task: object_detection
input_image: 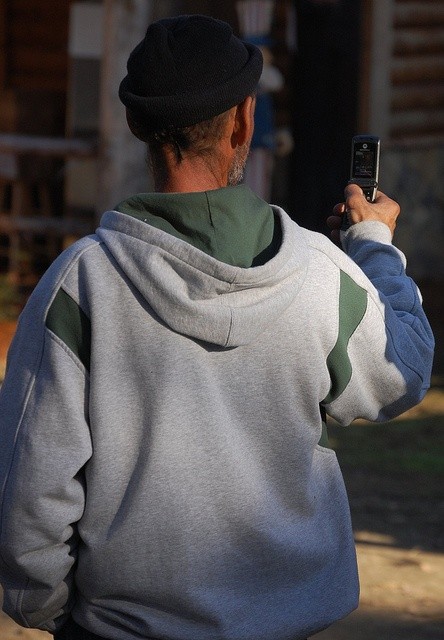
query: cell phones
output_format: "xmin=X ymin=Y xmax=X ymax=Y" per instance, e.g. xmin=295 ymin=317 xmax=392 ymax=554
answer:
xmin=342 ymin=136 xmax=381 ymax=232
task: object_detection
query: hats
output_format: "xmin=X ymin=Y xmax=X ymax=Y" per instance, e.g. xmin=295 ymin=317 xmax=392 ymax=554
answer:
xmin=118 ymin=15 xmax=264 ymax=126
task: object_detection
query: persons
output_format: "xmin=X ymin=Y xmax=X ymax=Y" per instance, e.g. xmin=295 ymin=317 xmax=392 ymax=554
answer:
xmin=0 ymin=12 xmax=436 ymax=638
